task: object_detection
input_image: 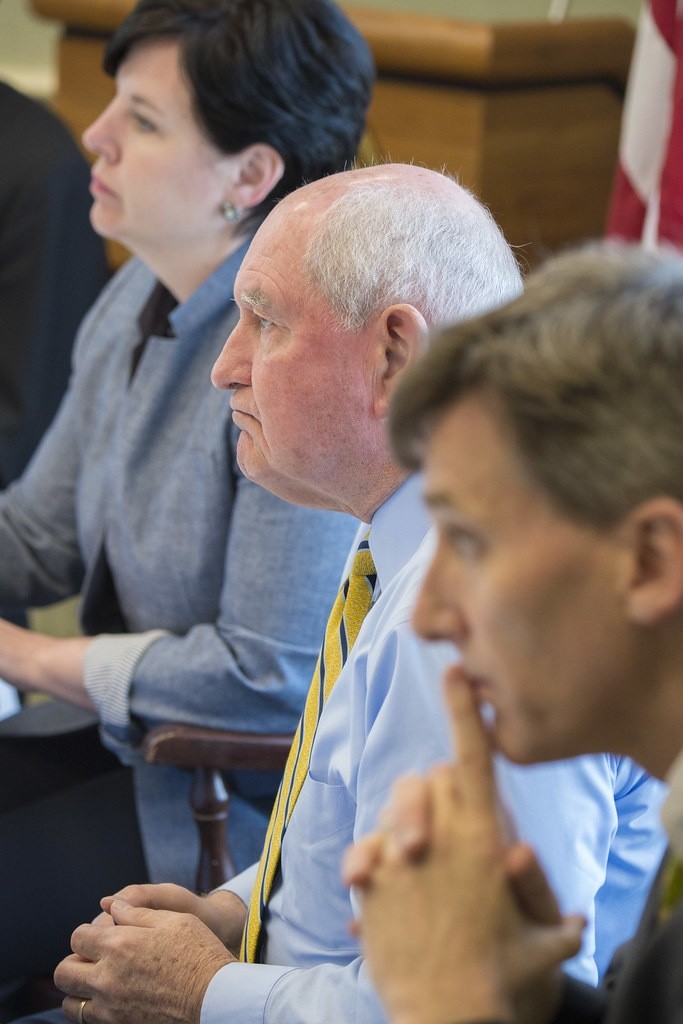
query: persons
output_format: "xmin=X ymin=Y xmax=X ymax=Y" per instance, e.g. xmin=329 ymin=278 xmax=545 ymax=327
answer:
xmin=338 ymin=238 xmax=683 ymax=1024
xmin=54 ymin=163 xmax=668 ymax=1024
xmin=0 ymin=0 xmax=377 ymax=1022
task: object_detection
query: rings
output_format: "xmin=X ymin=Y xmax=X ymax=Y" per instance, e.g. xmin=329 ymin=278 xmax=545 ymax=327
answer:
xmin=77 ymin=999 xmax=87 ymax=1024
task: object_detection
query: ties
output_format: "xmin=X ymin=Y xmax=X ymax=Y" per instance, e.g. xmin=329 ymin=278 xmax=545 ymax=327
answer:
xmin=233 ymin=542 xmax=379 ymax=961
